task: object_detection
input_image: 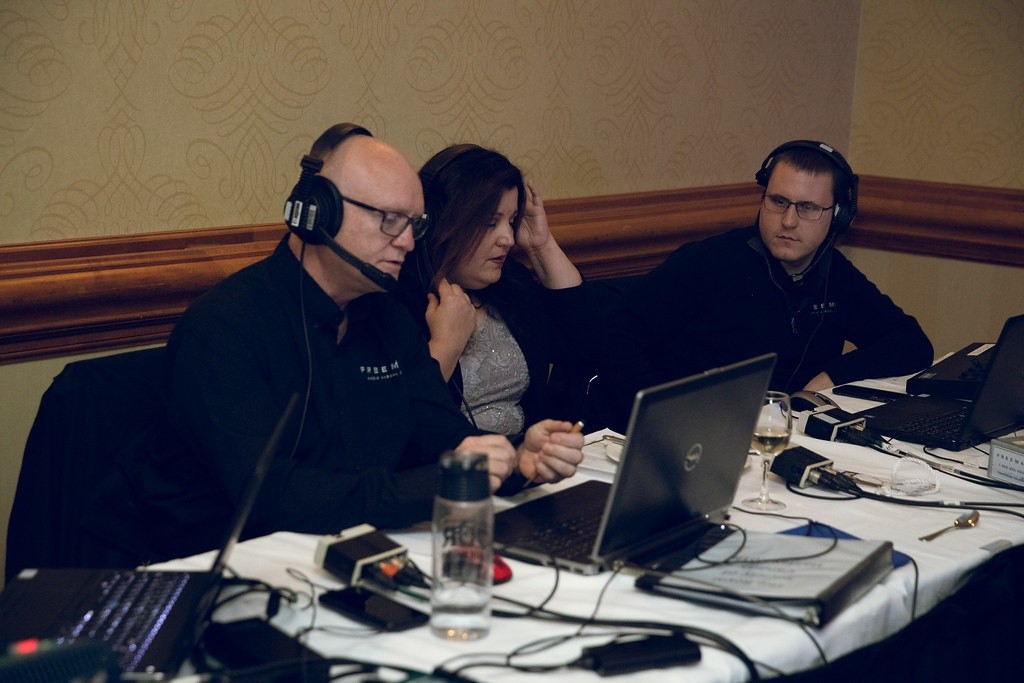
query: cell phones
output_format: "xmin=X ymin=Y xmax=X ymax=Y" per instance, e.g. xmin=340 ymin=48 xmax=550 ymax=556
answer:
xmin=319 ymin=584 xmax=429 ymax=632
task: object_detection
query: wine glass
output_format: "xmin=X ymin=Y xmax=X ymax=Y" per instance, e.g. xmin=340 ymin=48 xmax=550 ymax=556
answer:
xmin=741 ymin=390 xmax=793 ymax=513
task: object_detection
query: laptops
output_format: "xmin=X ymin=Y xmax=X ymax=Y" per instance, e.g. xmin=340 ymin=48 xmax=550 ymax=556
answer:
xmin=492 ymin=352 xmax=777 ymax=577
xmin=854 ymin=313 xmax=1024 ymax=451
xmin=0 ymin=398 xmax=304 ymax=682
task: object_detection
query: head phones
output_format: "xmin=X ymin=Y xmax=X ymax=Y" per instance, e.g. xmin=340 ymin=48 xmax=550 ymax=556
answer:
xmin=756 ymin=141 xmax=861 ymax=236
xmin=284 ymin=121 xmax=372 ymax=245
xmin=410 ymin=142 xmax=477 ymax=241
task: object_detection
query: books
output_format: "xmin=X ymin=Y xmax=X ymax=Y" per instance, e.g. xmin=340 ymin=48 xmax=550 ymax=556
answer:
xmin=635 ymin=527 xmax=893 ymax=632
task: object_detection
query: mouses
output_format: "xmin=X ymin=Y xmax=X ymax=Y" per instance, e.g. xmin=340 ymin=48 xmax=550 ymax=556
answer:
xmin=790 ymin=390 xmax=841 ymax=418
xmin=444 ymin=545 xmax=512 ymax=585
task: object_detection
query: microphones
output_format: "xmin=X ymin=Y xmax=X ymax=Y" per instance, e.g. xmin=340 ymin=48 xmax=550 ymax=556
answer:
xmin=318 ymin=226 xmax=398 ymax=292
xmin=421 ymin=240 xmax=440 ymax=300
xmin=792 ymin=231 xmax=838 ymax=285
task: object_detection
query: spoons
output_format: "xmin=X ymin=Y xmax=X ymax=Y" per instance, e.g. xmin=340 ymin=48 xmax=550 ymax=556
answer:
xmin=918 ymin=510 xmax=980 ymax=543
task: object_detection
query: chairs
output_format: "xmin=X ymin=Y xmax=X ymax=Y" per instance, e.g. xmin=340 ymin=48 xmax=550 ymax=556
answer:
xmin=3 ymin=347 xmax=183 ymax=594
xmin=545 ymin=273 xmax=646 ymax=436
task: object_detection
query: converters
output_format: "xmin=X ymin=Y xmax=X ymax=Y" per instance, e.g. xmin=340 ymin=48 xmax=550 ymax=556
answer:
xmin=202 ymin=616 xmax=330 ymax=682
xmin=582 ymin=636 xmax=701 ymax=676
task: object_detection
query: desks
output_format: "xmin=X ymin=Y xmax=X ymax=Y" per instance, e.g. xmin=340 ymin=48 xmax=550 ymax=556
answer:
xmin=133 ymin=350 xmax=1024 ymax=683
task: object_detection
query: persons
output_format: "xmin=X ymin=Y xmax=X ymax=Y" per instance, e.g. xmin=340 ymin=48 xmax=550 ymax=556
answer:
xmin=650 ymin=140 xmax=935 ymax=398
xmin=365 ymin=145 xmax=647 ymax=447
xmin=126 ymin=134 xmax=584 ymax=552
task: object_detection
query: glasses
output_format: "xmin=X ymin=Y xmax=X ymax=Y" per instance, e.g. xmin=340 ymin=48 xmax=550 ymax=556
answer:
xmin=761 ymin=187 xmax=834 ymax=220
xmin=342 ymin=195 xmax=429 ymax=240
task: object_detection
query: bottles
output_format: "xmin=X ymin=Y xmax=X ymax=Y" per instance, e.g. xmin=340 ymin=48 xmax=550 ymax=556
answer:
xmin=428 ymin=450 xmax=494 ymax=643
